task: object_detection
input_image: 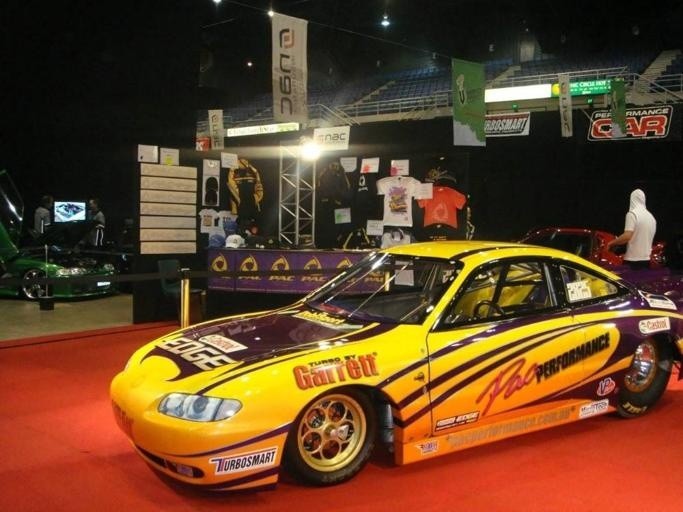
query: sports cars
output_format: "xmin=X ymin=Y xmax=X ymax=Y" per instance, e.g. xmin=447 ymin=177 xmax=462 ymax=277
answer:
xmin=105 ymin=239 xmax=682 ymax=494
xmin=0 ymin=169 xmax=119 ymax=301
xmin=501 ymin=226 xmax=667 ymax=275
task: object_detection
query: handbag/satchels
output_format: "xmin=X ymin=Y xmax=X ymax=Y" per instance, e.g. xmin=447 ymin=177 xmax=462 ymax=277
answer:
xmin=381 ymin=228 xmax=410 ymax=250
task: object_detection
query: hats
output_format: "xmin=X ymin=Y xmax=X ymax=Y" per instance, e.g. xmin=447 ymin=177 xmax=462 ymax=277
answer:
xmin=225 ymin=234 xmax=244 ymax=249
xmin=208 ymin=235 xmax=225 ymax=248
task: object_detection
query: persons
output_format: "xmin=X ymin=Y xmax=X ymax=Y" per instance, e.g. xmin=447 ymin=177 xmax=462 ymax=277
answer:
xmin=33 ymin=194 xmax=54 ymax=233
xmin=605 ymin=187 xmax=657 ymax=270
xmin=82 ymin=198 xmax=105 ymax=248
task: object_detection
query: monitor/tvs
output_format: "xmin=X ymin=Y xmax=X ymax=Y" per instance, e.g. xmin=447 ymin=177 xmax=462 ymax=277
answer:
xmin=54 ymin=201 xmax=87 ymax=223
